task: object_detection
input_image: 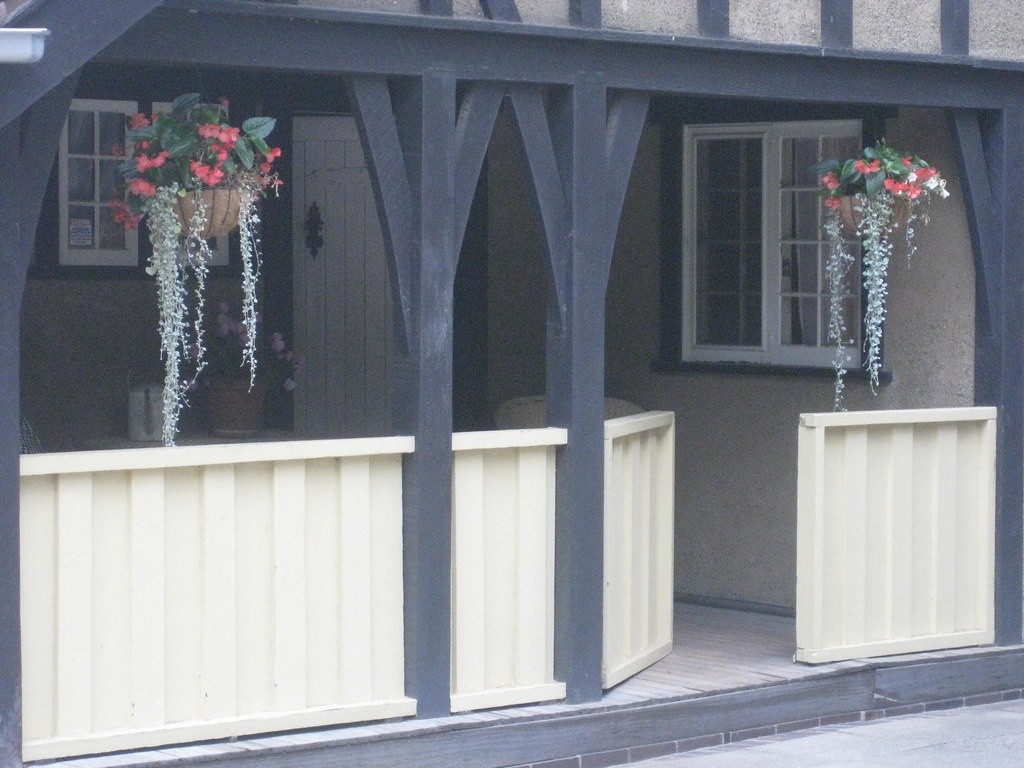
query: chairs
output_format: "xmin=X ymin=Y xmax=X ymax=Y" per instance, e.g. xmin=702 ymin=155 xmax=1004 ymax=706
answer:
xmin=493 ymin=395 xmax=647 ymax=431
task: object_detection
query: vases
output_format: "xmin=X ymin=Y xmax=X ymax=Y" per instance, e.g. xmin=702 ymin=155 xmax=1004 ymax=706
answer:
xmin=166 ymin=187 xmax=249 ymax=239
xmin=837 ymin=192 xmax=912 ymax=242
xmin=203 ymin=374 xmax=274 ymax=440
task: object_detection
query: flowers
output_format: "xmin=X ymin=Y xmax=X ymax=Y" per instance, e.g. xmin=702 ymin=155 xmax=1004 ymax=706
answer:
xmin=105 ymin=91 xmax=284 ymax=447
xmin=807 ymin=137 xmax=949 ymax=413
xmin=182 ymin=309 xmax=307 ymax=393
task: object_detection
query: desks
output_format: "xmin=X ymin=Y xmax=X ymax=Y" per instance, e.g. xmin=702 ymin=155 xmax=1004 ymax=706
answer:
xmin=86 ymin=425 xmax=316 ymax=451
